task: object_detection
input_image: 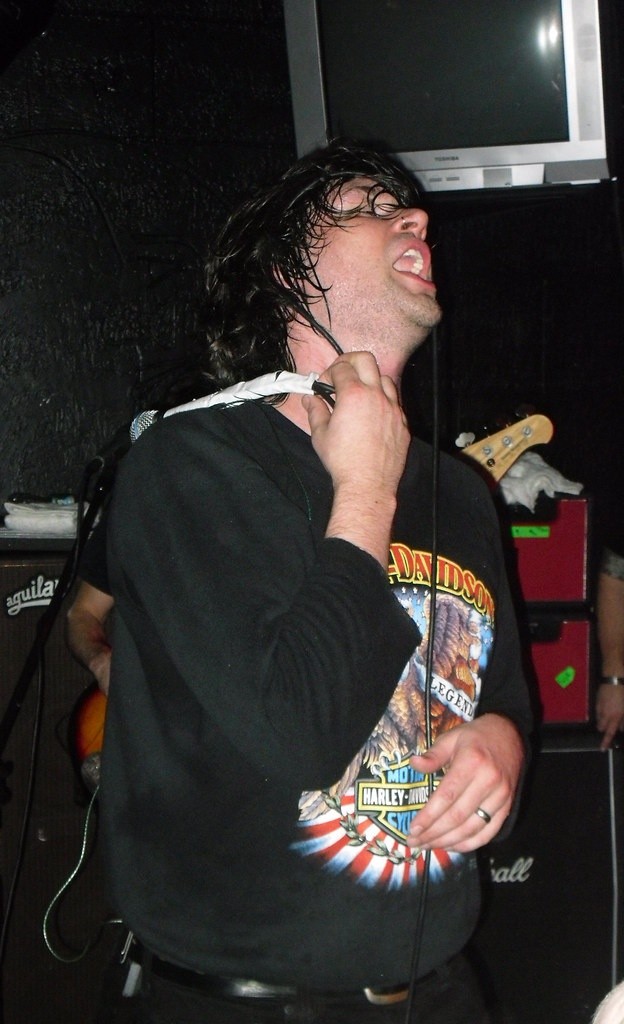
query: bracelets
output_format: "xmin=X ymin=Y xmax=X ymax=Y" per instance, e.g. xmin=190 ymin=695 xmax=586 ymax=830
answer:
xmin=600 ymin=677 xmax=624 ymax=685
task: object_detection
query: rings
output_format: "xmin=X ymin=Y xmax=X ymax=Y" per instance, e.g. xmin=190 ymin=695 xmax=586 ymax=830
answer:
xmin=475 ymin=808 xmax=491 ymax=824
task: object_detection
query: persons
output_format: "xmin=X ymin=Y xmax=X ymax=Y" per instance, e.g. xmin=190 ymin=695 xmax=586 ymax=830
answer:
xmin=66 ymin=137 xmax=544 ymax=1024
xmin=594 ymin=492 xmax=624 ymax=753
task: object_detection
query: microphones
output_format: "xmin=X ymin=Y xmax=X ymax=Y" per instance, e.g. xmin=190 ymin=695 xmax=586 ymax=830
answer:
xmin=89 ymin=423 xmax=132 ymax=473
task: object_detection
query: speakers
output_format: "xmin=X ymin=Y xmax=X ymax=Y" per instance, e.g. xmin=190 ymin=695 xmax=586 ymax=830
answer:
xmin=0 ymin=528 xmax=148 ymax=1024
xmin=466 ymin=722 xmax=624 ymax=1024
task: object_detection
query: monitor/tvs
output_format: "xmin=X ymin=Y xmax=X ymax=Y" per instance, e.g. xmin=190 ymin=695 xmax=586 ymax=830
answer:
xmin=284 ymin=0 xmax=624 ymax=194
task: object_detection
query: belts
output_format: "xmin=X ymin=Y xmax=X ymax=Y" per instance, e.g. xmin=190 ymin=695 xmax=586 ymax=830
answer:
xmin=127 ymin=944 xmax=438 ymax=1007
